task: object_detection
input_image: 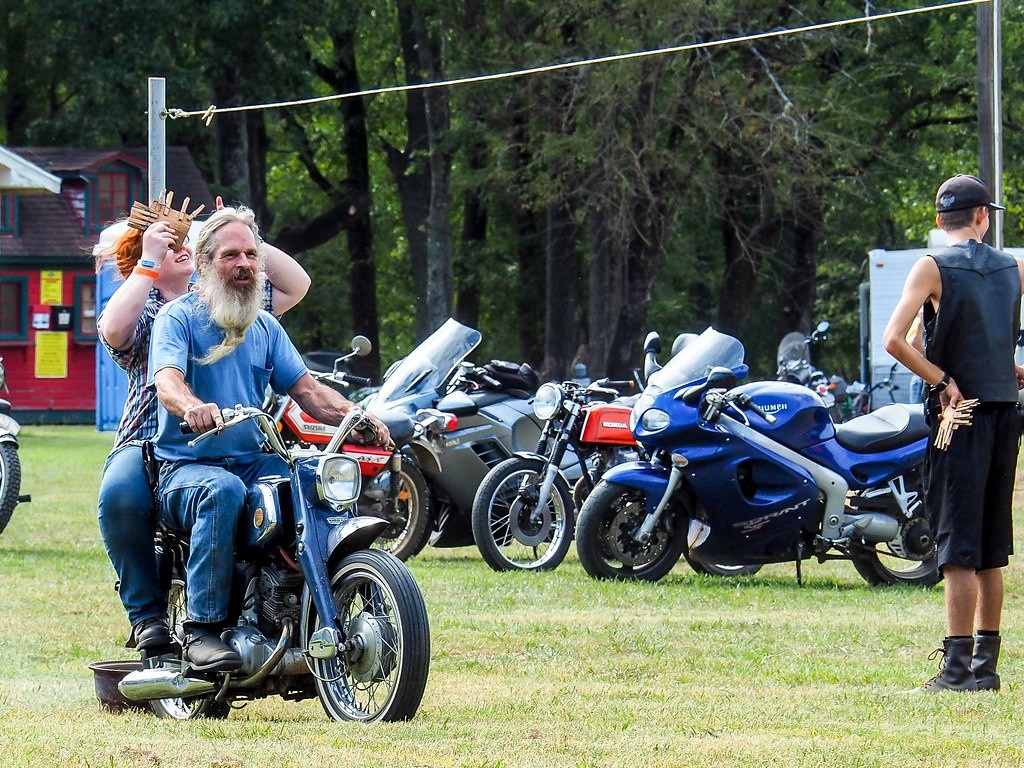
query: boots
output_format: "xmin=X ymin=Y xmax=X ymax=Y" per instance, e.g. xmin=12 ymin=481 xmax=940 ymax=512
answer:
xmin=894 ymin=636 xmax=1002 ymax=697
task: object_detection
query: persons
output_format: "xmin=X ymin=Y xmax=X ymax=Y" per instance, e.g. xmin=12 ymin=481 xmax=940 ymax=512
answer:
xmin=883 ymin=174 xmax=1024 ymax=695
xmin=97 ymin=195 xmax=311 ymax=650
xmin=146 ymin=207 xmax=395 ymax=669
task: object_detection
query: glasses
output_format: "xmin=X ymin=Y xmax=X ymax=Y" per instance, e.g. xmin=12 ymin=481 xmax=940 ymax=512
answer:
xmin=166 ymin=235 xmax=190 ymax=250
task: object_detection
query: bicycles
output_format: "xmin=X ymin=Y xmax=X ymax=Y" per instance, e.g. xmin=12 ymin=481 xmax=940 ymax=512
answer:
xmin=845 ymin=362 xmax=901 ymax=418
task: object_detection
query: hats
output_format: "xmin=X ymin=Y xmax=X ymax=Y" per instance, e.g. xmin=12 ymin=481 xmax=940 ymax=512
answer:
xmin=935 ymin=174 xmax=1006 ymax=213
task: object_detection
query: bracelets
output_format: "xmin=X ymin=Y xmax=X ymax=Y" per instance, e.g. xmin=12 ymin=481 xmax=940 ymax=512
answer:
xmin=937 ymin=373 xmax=950 ymax=392
xmin=133 ymin=266 xmax=159 ymax=281
xmin=137 ymin=259 xmax=161 ymax=268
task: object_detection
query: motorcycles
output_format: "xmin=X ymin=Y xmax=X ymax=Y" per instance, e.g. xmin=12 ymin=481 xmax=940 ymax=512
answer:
xmin=775 ymin=322 xmax=842 ymax=425
xmin=261 ymin=317 xmax=644 ymax=563
xmin=575 ymin=325 xmax=944 ymax=590
xmin=470 ymin=377 xmax=766 ymax=578
xmin=0 ymin=358 xmax=31 ymax=535
xmin=117 ymin=403 xmax=431 ymax=724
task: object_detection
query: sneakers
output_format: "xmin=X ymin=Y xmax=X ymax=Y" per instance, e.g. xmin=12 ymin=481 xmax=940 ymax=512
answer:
xmin=125 ymin=617 xmax=170 ymax=652
xmin=181 ymin=620 xmax=243 ymax=678
